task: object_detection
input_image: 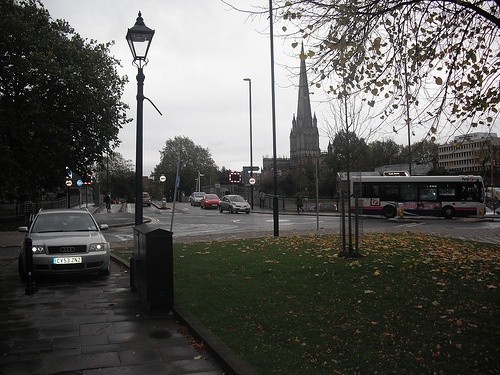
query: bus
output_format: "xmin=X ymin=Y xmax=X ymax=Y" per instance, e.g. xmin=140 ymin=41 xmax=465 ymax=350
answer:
xmin=348 ymin=170 xmax=486 ymax=218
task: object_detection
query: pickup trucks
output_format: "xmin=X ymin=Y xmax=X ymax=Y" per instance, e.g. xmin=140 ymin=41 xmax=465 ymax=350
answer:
xmin=141 ymin=191 xmax=151 ymax=205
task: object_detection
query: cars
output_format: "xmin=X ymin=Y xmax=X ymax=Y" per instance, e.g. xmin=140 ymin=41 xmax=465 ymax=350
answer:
xmin=201 ymin=193 xmax=220 ymax=209
xmin=17 ymin=206 xmax=112 ymax=283
xmin=219 ymin=195 xmax=251 ymax=214
xmin=190 ymin=192 xmax=206 ymax=206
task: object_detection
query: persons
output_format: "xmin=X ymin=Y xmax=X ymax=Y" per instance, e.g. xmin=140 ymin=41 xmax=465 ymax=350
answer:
xmin=296 ymin=194 xmax=304 ymax=214
xmin=259 ymin=190 xmax=265 ymax=208
xmin=106 ymin=193 xmax=112 ymax=213
xmin=23 ymin=197 xmax=33 ymax=228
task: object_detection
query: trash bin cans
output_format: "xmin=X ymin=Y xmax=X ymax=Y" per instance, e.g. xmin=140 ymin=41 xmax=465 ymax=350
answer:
xmin=162 ymin=197 xmax=166 ymax=207
xmin=129 ymin=222 xmax=174 ymax=305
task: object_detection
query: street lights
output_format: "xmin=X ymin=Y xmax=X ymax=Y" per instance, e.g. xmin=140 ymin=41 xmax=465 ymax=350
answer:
xmin=126 ymin=9 xmax=156 ymax=289
xmin=243 ymin=77 xmax=254 ymax=209
